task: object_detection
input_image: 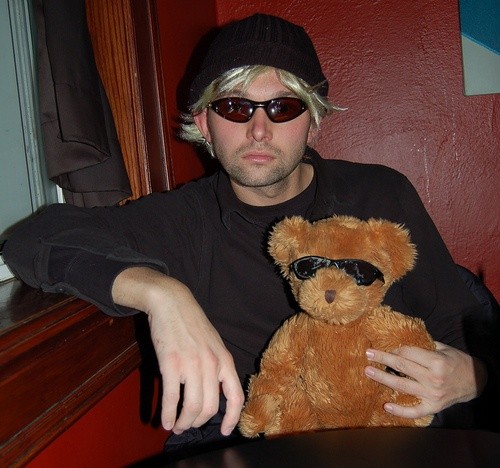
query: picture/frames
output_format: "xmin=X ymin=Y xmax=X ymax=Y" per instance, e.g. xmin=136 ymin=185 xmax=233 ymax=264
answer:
xmin=457 ymin=0 xmax=500 ymax=96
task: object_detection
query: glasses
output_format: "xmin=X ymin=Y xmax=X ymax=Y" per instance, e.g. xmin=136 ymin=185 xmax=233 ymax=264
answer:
xmin=207 ymin=97 xmax=308 ymax=123
xmin=289 ymin=256 xmax=387 ymax=287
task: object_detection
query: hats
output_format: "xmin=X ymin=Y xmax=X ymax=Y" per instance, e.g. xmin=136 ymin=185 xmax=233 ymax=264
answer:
xmin=177 ymin=14 xmax=328 ymax=114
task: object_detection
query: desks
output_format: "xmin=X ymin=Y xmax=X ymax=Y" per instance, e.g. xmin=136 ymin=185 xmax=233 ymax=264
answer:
xmin=123 ymin=427 xmax=500 ymax=467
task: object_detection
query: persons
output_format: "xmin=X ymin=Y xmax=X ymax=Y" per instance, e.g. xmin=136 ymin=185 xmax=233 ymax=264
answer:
xmin=0 ymin=13 xmax=499 ymax=449
